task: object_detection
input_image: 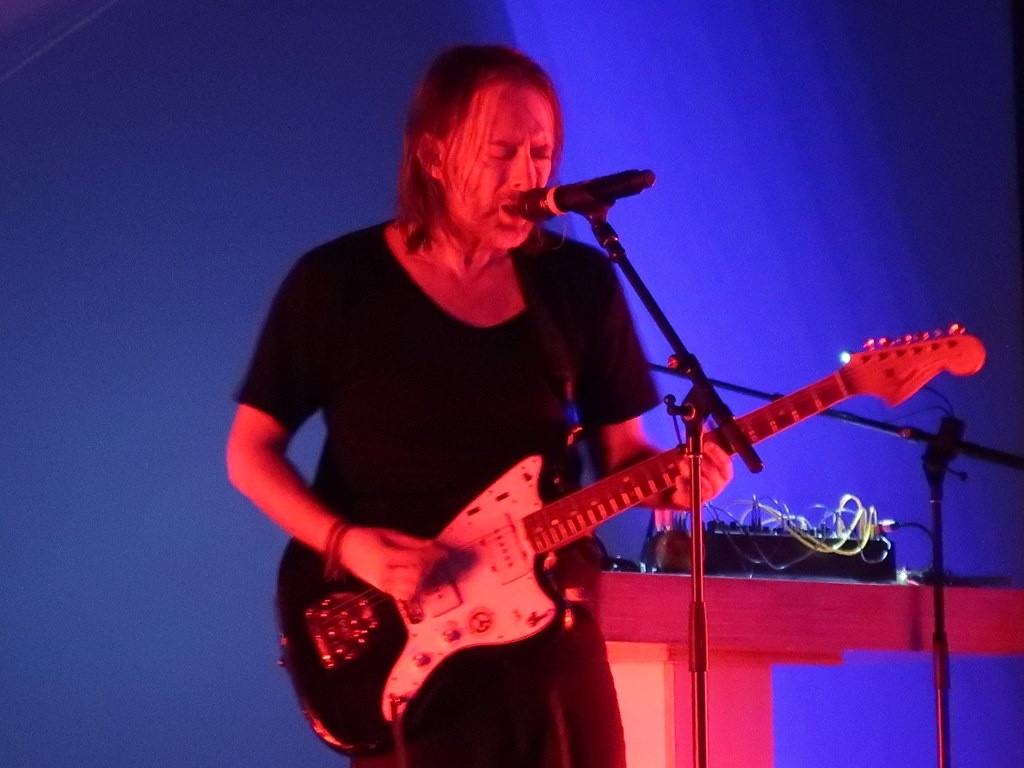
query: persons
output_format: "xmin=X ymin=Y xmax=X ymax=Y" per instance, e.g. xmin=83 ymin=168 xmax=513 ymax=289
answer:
xmin=226 ymin=41 xmax=735 ymax=768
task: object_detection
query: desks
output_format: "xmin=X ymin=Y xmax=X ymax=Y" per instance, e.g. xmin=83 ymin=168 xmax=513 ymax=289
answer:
xmin=597 ymin=570 xmax=1024 ymax=768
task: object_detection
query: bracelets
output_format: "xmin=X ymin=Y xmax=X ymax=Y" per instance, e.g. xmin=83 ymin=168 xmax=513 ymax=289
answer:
xmin=322 ymin=518 xmax=353 ymax=562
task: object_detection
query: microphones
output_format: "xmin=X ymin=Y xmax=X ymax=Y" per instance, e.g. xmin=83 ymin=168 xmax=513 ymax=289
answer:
xmin=518 ymin=169 xmax=657 ymax=224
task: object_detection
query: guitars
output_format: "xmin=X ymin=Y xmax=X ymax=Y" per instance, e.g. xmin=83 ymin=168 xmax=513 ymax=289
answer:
xmin=274 ymin=324 xmax=988 ymax=755
